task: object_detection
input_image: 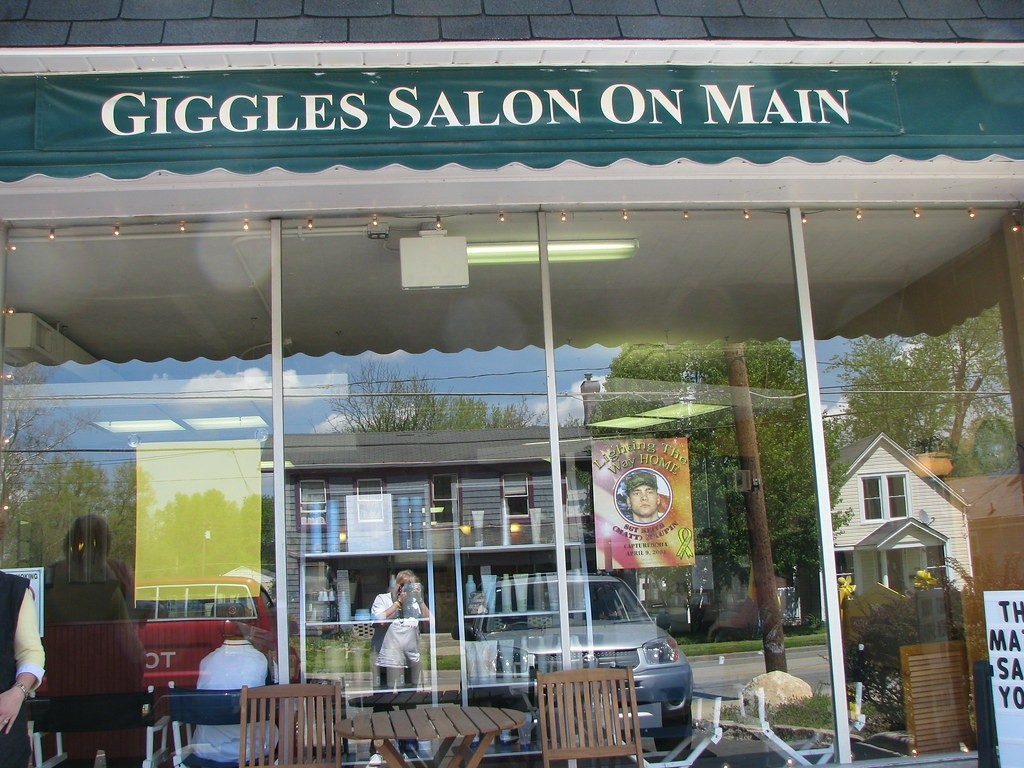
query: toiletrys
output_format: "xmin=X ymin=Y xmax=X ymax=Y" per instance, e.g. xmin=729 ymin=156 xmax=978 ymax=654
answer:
xmin=464 ymin=497 xmax=584 ymax=752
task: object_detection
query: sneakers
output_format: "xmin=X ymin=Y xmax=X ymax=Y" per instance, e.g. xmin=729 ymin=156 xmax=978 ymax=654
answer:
xmin=369 ymin=750 xmax=385 ymax=765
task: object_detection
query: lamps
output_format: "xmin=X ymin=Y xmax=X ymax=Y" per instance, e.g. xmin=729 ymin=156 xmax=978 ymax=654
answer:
xmin=468 ymin=238 xmax=641 ymax=265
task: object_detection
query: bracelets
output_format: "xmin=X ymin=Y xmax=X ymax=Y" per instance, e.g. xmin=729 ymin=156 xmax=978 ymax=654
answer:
xmin=417 ymin=598 xmax=423 ymax=604
xmin=394 ymin=599 xmax=402 ymax=608
xmin=14 ymin=682 xmax=29 ymax=701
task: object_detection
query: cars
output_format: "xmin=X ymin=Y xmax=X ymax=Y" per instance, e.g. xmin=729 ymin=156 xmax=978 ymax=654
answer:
xmin=451 ymin=576 xmax=692 ymax=762
xmin=124 ymin=577 xmax=299 ymax=749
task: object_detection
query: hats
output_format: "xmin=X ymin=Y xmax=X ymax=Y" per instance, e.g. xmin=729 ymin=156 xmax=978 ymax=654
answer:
xmin=626 ymin=472 xmax=658 ymax=491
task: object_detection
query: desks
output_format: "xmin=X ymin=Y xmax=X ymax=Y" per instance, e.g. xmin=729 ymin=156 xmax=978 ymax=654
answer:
xmin=336 ymin=705 xmax=527 ymax=768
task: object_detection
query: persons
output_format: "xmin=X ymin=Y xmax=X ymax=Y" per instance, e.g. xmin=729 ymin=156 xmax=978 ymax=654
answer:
xmin=0 ymin=510 xmax=45 ymax=768
xmin=366 ymin=569 xmax=430 ymax=766
xmin=41 ymin=514 xmax=150 ymax=768
xmin=180 ymin=600 xmax=279 ymax=768
xmin=626 ymin=473 xmax=661 ymax=524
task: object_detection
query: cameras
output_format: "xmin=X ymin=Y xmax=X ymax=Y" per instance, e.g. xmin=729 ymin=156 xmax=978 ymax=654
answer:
xmin=403 ymin=583 xmax=417 ymax=592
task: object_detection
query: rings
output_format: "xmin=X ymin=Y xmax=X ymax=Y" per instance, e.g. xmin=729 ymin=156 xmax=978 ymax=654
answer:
xmin=2 ymin=720 xmax=8 ymax=725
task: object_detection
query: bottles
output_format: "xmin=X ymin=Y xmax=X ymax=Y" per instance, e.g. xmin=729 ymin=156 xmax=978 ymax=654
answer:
xmin=466 ymin=575 xmax=477 ymax=615
xmin=519 ymin=635 xmax=530 ymax=678
xmin=306 ymin=590 xmax=340 ymax=622
xmin=533 ymin=573 xmax=545 ymax=611
xmin=573 ymin=569 xmax=585 ymax=610
xmin=538 ymin=636 xmax=548 ymax=673
xmin=501 ymin=574 xmax=512 ymax=614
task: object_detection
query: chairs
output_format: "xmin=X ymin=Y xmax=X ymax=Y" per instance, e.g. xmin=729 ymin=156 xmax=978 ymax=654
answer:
xmin=535 ymin=667 xmax=644 ymax=768
xmin=239 ymin=683 xmax=342 ymax=767
xmin=167 ymin=680 xmax=278 ymax=768
xmin=25 ymin=685 xmax=171 ymax=768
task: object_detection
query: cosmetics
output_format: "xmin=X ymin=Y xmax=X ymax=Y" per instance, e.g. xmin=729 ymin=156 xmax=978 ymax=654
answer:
xmin=354 ymin=609 xmax=369 ymax=620
xmin=464 ymin=497 xmax=588 ymax=755
